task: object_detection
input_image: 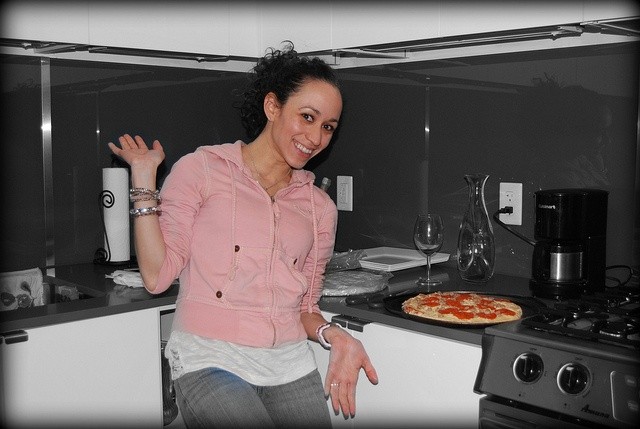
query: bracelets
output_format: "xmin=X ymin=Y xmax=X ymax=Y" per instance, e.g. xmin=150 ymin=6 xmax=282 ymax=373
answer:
xmin=129 ymin=206 xmax=162 ymax=217
xmin=129 ymin=187 xmax=161 ymax=204
xmin=316 ymin=321 xmax=337 ymax=349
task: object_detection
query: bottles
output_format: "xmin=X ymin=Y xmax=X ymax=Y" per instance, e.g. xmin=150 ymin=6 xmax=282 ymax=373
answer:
xmin=456 ymin=172 xmax=496 ymax=283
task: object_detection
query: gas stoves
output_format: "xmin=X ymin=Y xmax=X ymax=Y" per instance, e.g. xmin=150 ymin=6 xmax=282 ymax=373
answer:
xmin=471 ymin=281 xmax=639 ymax=429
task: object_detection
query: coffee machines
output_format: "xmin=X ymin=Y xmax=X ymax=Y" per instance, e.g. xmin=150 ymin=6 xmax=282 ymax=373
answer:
xmin=526 ymin=187 xmax=608 ymax=302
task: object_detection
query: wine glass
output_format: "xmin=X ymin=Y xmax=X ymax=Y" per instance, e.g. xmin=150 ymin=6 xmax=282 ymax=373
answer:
xmin=414 ymin=212 xmax=444 ymax=286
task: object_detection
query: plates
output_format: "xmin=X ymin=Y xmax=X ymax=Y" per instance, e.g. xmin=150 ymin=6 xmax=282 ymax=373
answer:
xmin=381 ymin=289 xmax=549 ymax=332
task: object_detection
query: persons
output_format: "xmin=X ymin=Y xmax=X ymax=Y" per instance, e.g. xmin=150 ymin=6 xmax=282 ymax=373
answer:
xmin=109 ymin=40 xmax=378 ymax=427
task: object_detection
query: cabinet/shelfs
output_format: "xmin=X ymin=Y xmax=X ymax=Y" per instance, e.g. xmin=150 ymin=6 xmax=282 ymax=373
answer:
xmin=0 ymin=307 xmax=169 ymax=428
xmin=308 ymin=306 xmax=483 ymax=429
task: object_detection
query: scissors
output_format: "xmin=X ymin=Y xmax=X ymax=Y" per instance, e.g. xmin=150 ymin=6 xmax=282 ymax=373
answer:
xmin=344 ymin=288 xmax=411 ymax=309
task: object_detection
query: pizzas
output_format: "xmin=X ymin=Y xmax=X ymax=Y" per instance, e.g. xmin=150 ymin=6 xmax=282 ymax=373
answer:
xmin=401 ymin=290 xmax=523 ymax=325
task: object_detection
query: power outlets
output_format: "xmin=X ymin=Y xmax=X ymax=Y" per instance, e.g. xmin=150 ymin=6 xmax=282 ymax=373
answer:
xmin=499 ymin=182 xmax=522 ymax=226
xmin=337 ymin=175 xmax=353 ymax=212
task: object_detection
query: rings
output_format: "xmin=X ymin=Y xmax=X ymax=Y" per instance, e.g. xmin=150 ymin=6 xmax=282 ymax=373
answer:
xmin=331 ymin=384 xmax=339 ymax=387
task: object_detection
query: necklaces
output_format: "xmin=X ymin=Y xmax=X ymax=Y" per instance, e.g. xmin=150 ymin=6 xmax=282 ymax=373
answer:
xmin=246 ymin=145 xmax=292 ymax=190
